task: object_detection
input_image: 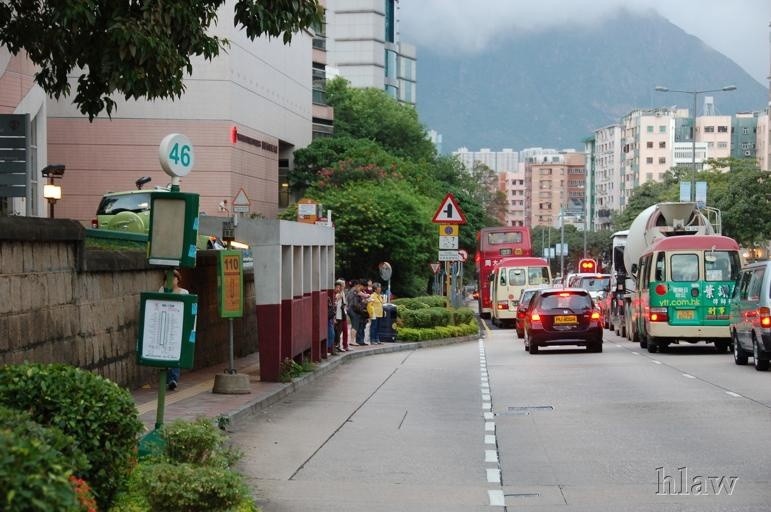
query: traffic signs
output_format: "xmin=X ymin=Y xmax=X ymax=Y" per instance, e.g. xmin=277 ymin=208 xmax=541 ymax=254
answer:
xmin=214 ymin=248 xmax=244 ymax=319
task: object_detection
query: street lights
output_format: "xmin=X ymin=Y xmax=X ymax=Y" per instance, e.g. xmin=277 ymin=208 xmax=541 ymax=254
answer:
xmin=654 ymin=85 xmax=735 ymax=199
xmin=536 ymin=212 xmax=574 ymax=274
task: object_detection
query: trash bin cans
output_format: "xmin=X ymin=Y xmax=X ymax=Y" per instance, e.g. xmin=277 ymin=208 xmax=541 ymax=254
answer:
xmin=378 ymin=303 xmax=398 ymax=343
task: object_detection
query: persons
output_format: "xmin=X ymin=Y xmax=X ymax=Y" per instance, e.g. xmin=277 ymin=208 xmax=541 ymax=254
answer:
xmin=158 ymin=270 xmax=189 ymax=390
xmin=329 ymin=277 xmax=389 ymax=355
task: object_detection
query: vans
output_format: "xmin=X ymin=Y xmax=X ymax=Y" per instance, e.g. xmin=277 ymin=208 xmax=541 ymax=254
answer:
xmin=488 ymin=258 xmax=552 ymax=329
xmin=629 ymin=236 xmax=743 ymax=354
xmin=726 ymin=260 xmax=769 ymax=371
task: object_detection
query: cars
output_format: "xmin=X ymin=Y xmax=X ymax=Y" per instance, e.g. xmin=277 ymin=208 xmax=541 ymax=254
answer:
xmin=514 ymin=288 xmax=602 ymax=354
xmin=556 ymin=273 xmax=610 ymax=329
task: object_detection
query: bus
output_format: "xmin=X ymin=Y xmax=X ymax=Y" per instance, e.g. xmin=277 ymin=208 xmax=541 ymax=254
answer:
xmin=472 ymin=227 xmax=533 ymax=318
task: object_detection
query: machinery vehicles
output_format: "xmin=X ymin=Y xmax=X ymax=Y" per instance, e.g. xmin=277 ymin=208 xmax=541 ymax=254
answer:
xmin=610 ymin=201 xmax=722 ymax=342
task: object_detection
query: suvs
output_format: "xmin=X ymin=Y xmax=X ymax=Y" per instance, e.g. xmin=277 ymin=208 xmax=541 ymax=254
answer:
xmin=90 ymin=189 xmax=171 ymax=249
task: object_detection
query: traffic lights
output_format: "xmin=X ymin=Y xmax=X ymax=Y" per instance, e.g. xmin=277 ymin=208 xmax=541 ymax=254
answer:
xmin=578 ymin=259 xmax=596 ymax=273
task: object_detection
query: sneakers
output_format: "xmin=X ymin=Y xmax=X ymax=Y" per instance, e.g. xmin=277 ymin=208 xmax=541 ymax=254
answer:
xmin=336 ymin=341 xmax=384 ymax=352
